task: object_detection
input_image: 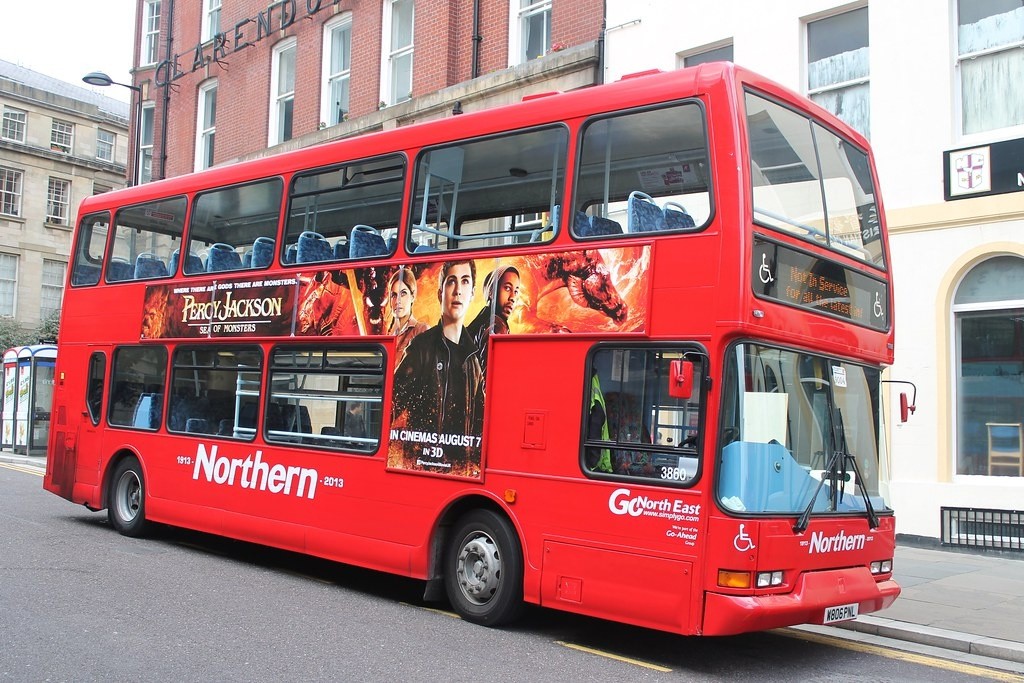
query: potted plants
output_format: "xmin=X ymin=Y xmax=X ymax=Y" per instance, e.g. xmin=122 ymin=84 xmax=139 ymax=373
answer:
xmin=408 ymin=91 xmax=412 ymax=100
xmin=318 ymin=122 xmax=326 ymax=130
xmin=378 ymin=101 xmax=386 ymax=110
xmin=344 ymin=115 xmax=349 ymax=122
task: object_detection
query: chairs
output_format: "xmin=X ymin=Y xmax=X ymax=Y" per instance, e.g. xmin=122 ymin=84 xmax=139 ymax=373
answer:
xmin=74 ymin=190 xmax=695 ymax=288
xmin=90 ymin=377 xmax=344 ymax=452
xmin=603 ymin=393 xmax=650 ymax=471
xmin=984 ymin=422 xmax=1024 ymax=476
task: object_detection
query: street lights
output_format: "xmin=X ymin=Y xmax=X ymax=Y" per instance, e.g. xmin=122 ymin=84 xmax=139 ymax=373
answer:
xmin=82 ymin=71 xmax=146 ymax=264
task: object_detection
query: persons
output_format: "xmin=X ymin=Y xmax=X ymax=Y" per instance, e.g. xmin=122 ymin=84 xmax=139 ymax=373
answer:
xmin=462 ymin=265 xmax=520 ymax=379
xmin=382 ymin=269 xmax=428 ymax=370
xmin=344 ymin=401 xmax=368 ymax=439
xmin=391 ymin=260 xmax=488 ymax=478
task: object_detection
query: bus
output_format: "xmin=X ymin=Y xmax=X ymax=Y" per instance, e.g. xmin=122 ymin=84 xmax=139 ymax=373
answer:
xmin=41 ymin=59 xmax=918 ymax=631
